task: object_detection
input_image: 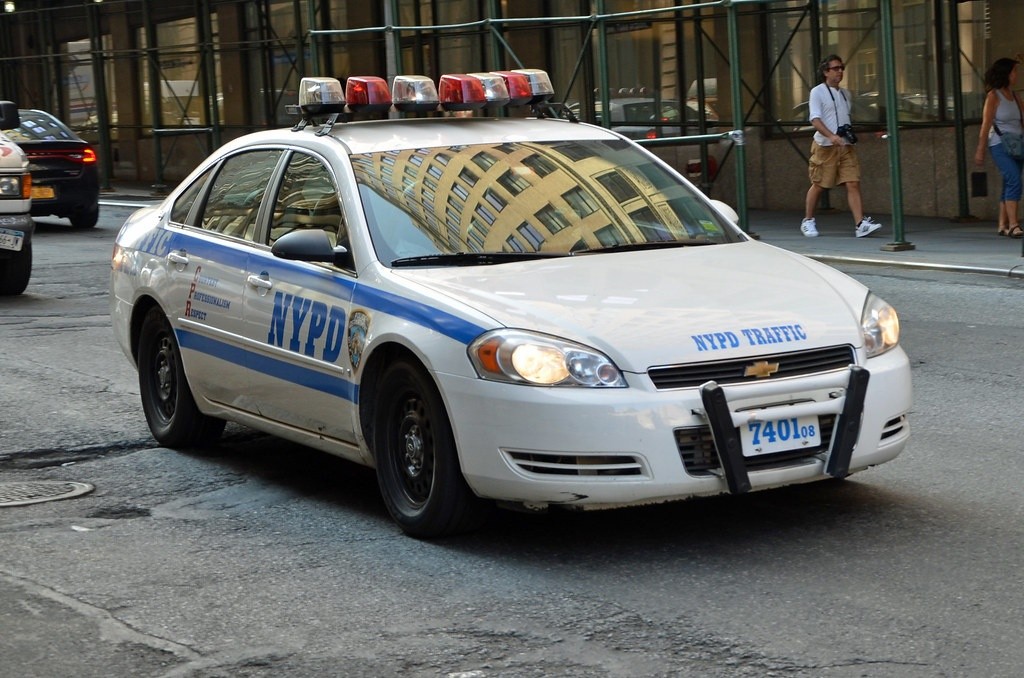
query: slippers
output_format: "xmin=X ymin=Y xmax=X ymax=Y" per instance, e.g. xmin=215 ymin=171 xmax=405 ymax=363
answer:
xmin=997 ymin=226 xmax=1009 ymax=236
xmin=1009 ymin=226 xmax=1024 ymax=238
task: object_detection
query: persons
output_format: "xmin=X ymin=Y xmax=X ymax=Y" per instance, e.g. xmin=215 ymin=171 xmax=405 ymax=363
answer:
xmin=800 ymin=55 xmax=882 ymax=238
xmin=974 ymin=57 xmax=1024 ymax=239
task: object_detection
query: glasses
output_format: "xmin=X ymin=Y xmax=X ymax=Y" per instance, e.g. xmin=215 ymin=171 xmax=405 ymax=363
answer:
xmin=828 ymin=65 xmax=845 ymax=72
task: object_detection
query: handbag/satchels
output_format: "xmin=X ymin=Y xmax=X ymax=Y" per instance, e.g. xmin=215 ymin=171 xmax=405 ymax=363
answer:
xmin=999 ymin=131 xmax=1024 ymax=159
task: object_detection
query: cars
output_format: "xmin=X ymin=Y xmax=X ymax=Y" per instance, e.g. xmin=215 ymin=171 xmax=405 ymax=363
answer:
xmin=0 ymin=98 xmax=34 ymax=298
xmin=0 ymin=110 xmax=102 ymax=229
xmin=109 ymin=69 xmax=915 ymax=539
xmin=82 ymin=55 xmax=991 ymax=126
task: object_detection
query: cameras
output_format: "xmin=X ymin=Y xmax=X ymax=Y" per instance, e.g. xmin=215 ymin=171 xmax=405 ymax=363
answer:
xmin=836 ymin=124 xmax=858 ymax=144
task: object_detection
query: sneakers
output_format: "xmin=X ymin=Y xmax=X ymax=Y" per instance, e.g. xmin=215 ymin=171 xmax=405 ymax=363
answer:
xmin=801 ymin=217 xmax=819 ymax=237
xmin=855 ymin=215 xmax=882 ymax=237
